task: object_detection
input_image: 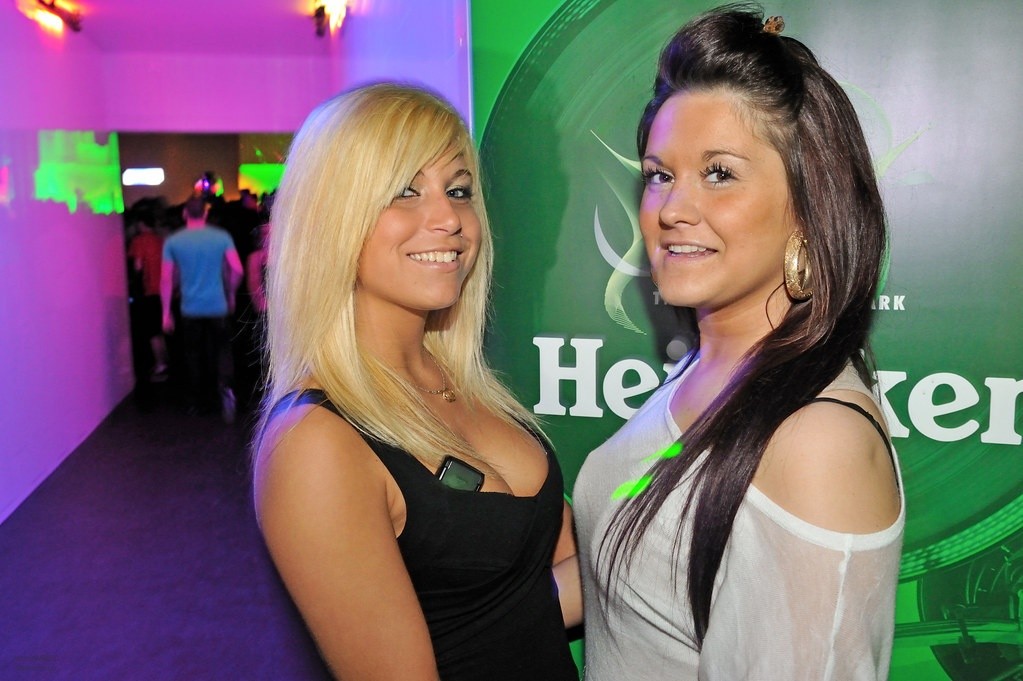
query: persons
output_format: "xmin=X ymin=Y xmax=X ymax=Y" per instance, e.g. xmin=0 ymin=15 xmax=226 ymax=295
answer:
xmin=160 ymin=195 xmax=243 ymax=421
xmin=128 ymin=215 xmax=174 ymax=374
xmin=251 ymin=78 xmax=576 ymax=679
xmin=555 ymin=0 xmax=905 ymax=680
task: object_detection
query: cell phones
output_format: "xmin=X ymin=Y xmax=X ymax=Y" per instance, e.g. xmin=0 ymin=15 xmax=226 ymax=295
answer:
xmin=436 ymin=455 xmax=485 ymax=492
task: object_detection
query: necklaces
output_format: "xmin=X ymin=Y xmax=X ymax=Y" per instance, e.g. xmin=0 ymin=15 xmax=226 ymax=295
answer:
xmin=409 ymin=346 xmax=457 ymax=403
